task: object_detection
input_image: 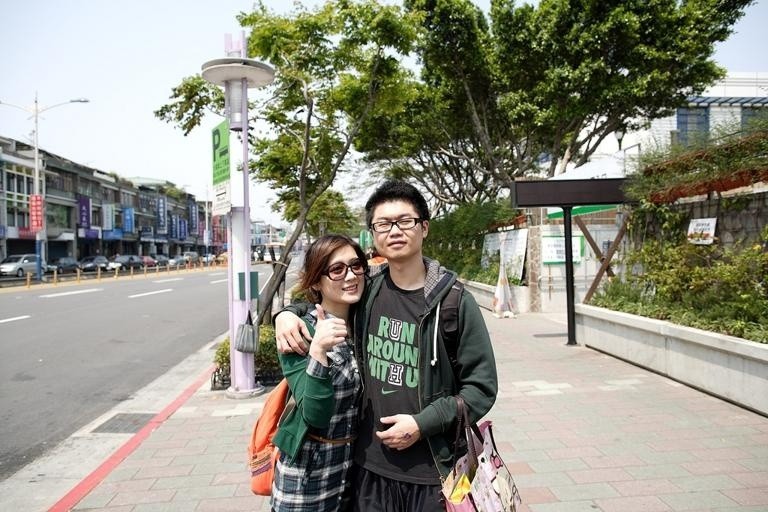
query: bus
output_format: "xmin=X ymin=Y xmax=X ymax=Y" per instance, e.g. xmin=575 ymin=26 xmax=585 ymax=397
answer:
xmin=261 ymin=240 xmax=286 ymax=263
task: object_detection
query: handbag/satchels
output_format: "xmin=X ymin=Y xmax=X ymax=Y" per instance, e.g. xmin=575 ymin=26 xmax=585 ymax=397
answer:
xmin=441 ymin=395 xmax=522 ymax=512
xmin=234 ymin=310 xmax=259 ymax=353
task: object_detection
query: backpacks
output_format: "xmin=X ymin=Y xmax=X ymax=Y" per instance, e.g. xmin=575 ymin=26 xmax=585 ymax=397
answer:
xmin=248 ymin=336 xmax=310 ymax=496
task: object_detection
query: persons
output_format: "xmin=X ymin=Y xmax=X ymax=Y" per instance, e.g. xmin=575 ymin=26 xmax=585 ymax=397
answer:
xmin=270 ymin=180 xmax=499 ymax=512
xmin=270 ymin=234 xmax=372 ymax=512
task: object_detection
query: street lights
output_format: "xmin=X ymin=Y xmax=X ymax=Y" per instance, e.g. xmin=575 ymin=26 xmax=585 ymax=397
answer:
xmin=0 ymin=91 xmax=90 ymax=274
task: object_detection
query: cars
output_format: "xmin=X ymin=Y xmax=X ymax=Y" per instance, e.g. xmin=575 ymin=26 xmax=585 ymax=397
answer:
xmin=0 ymin=251 xmax=230 ymax=280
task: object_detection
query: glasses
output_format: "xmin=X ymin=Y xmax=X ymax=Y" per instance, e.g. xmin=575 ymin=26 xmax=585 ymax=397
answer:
xmin=321 ymin=257 xmax=368 ymax=281
xmin=370 ymin=218 xmax=423 ymax=233
